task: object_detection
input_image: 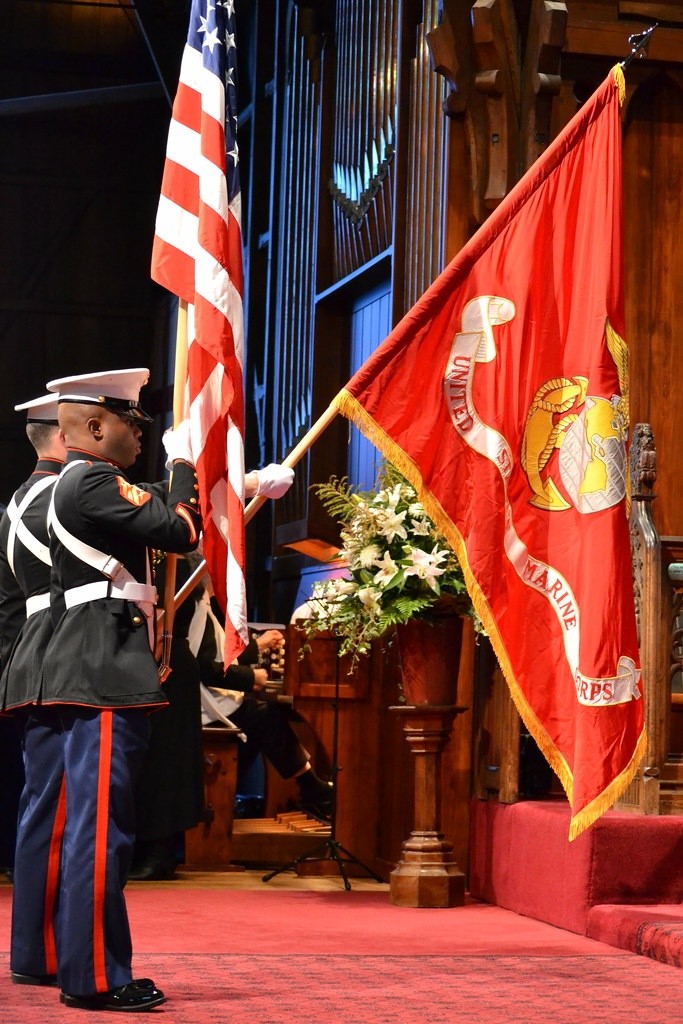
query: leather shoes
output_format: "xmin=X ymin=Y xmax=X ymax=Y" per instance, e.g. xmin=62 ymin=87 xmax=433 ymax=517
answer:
xmin=11 ymin=971 xmax=61 ymax=986
xmin=60 ymin=977 xmax=168 ymax=1011
xmin=126 ymin=866 xmax=169 ymax=881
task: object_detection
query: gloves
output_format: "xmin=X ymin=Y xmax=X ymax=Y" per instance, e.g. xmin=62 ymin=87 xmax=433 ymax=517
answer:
xmin=250 ymin=463 xmax=295 ymax=499
xmin=163 ymin=419 xmax=196 ymax=471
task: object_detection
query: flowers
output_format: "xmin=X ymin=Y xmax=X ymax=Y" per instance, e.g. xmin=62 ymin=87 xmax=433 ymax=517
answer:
xmin=297 ymin=451 xmax=469 ymax=677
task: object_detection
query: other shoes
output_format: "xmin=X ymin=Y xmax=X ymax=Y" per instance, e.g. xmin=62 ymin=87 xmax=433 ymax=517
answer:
xmin=300 ymin=781 xmax=335 ymax=813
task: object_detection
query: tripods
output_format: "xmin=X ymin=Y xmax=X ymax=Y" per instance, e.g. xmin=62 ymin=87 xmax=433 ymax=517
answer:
xmin=261 ymin=561 xmax=386 ymax=890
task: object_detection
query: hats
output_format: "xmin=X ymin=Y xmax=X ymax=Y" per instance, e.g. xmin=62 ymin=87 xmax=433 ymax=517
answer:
xmin=46 ymin=368 xmax=154 ymax=423
xmin=15 ymin=392 xmax=59 ymax=427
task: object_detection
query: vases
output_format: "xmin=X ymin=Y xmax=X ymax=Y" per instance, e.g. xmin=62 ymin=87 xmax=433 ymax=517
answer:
xmin=396 ymin=614 xmax=458 ymax=707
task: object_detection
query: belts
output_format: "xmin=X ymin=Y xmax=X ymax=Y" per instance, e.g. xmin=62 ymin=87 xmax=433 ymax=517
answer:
xmin=64 ymin=576 xmax=159 ymax=611
xmin=26 ymin=591 xmax=50 ymax=620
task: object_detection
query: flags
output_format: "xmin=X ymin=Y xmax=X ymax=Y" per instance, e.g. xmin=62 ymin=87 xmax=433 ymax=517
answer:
xmin=337 ymin=63 xmax=647 ymax=843
xmin=149 ymin=0 xmax=249 ymax=675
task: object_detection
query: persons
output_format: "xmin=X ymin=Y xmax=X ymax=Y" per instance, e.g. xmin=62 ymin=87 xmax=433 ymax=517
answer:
xmin=0 ymin=394 xmax=294 ymax=984
xmin=187 ymin=559 xmax=335 ymax=820
xmin=29 ymin=368 xmax=203 ymax=1010
xmin=123 ymin=670 xmax=199 ymax=883
